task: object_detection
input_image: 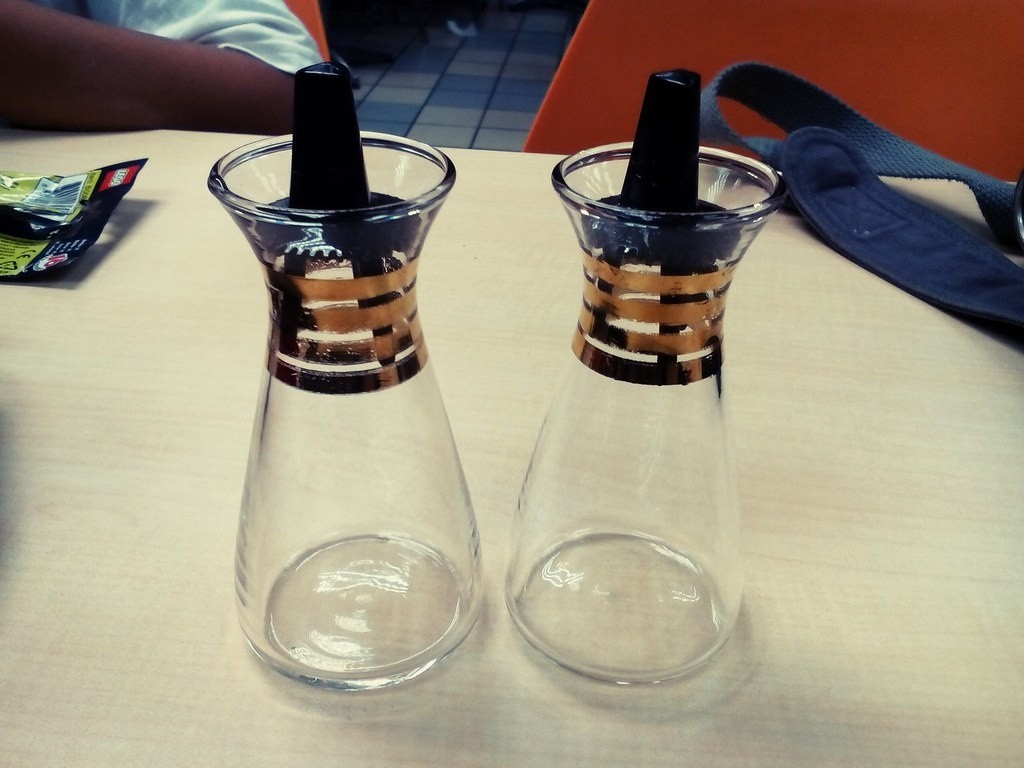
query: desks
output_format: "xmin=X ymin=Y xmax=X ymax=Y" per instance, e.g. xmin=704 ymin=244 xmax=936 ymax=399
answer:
xmin=0 ymin=129 xmax=1024 ymax=768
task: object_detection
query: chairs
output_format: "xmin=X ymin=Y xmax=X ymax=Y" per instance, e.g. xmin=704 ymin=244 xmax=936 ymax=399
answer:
xmin=520 ymin=0 xmax=1024 ymax=182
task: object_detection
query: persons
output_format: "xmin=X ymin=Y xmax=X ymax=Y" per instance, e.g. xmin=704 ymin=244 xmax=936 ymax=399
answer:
xmin=0 ymin=0 xmax=321 ymax=135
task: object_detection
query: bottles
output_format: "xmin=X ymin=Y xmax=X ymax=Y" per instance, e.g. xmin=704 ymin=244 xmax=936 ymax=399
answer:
xmin=206 ymin=58 xmax=488 ymax=696
xmin=503 ymin=67 xmax=790 ymax=682
